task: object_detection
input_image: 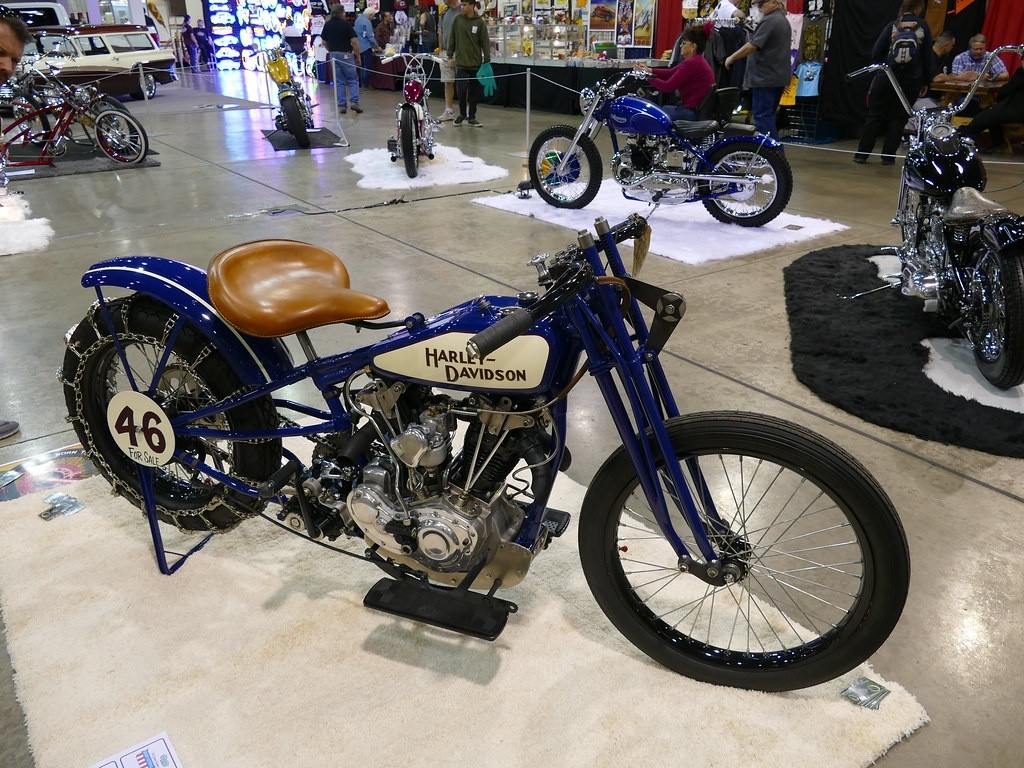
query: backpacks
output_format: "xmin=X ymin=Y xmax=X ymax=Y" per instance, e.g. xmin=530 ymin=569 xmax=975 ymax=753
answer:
xmin=887 ymin=19 xmax=922 ymax=83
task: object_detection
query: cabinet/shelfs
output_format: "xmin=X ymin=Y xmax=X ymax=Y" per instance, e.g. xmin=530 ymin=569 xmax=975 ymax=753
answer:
xmin=487 ymin=24 xmax=589 ymax=60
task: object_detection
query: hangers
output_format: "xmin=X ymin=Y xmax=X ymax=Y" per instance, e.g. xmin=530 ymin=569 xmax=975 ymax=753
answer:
xmin=695 ymin=18 xmax=736 ymax=30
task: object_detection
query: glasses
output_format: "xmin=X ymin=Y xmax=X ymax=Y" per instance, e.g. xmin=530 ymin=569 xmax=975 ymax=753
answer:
xmin=0 ymin=5 xmax=23 ymax=20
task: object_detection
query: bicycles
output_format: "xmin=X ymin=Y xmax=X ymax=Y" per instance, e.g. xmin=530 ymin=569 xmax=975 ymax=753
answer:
xmin=0 ymin=50 xmax=149 ymax=196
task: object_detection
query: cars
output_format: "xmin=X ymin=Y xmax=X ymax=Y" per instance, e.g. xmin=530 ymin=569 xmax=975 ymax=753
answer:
xmin=0 ymin=25 xmax=179 ymax=117
xmin=1 ymin=2 xmax=71 ymax=28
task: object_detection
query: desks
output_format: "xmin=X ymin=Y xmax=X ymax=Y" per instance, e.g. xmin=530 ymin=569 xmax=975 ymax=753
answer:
xmin=931 ymin=81 xmax=1013 ymax=154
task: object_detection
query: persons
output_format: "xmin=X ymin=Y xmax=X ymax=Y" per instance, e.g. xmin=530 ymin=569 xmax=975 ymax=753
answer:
xmin=181 ymin=15 xmax=201 ymax=73
xmin=436 ymin=0 xmax=462 ymax=121
xmin=192 ymin=20 xmax=215 ymax=70
xmin=633 ymin=25 xmax=716 ymax=121
xmin=446 ymin=0 xmax=490 ymax=126
xmin=374 ymin=12 xmax=394 ymax=48
xmin=69 ymin=13 xmax=87 ymax=24
xmin=282 ymin=18 xmax=302 ymax=72
xmin=355 ymin=7 xmax=382 ymax=90
xmin=320 ymin=4 xmax=363 ymax=113
xmin=923 ymin=31 xmax=1024 ymax=152
xmin=723 ymin=0 xmax=791 ymax=154
xmin=413 ymin=5 xmax=438 ymax=53
xmin=0 ymin=16 xmax=35 ymax=435
xmin=854 ymin=0 xmax=932 ymax=167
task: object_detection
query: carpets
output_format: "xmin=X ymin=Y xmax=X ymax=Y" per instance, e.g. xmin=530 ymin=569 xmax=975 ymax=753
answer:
xmin=343 ymin=141 xmax=508 ymax=190
xmin=471 ymin=176 xmax=851 ymax=265
xmin=0 ymin=139 xmax=162 ymax=257
xmin=262 ymin=127 xmax=351 ymax=152
xmin=1 ymin=391 xmax=932 ymax=768
xmin=783 ymin=245 xmax=1024 ymax=460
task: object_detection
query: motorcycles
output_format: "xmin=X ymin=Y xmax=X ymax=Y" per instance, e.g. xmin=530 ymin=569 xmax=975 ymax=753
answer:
xmin=528 ymin=71 xmax=793 ymax=228
xmin=379 ymin=53 xmax=448 ymax=179
xmin=57 ymin=215 xmax=913 ymax=692
xmin=250 ymin=43 xmax=320 ymax=148
xmin=836 ymin=44 xmax=1024 ymax=390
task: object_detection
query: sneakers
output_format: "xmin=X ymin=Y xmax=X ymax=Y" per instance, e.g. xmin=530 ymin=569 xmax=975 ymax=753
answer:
xmin=437 ymin=111 xmax=454 ymax=120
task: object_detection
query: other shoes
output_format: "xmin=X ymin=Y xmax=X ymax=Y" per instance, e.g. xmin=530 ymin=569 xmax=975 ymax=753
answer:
xmin=468 ymin=118 xmax=483 ymax=126
xmin=0 ymin=420 xmax=19 ymax=438
xmin=339 ymin=106 xmax=346 ymax=112
xmin=880 ymin=159 xmax=895 ymax=166
xmin=454 ymin=115 xmax=466 ymax=126
xmin=853 ymin=157 xmax=866 ymax=164
xmin=351 ymin=103 xmax=363 ymax=113
